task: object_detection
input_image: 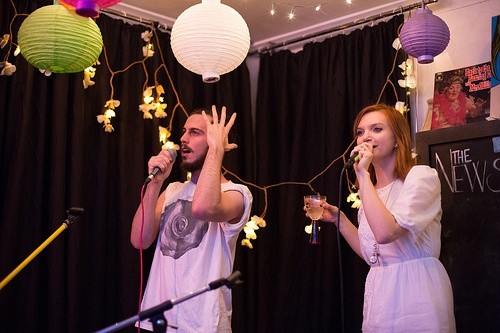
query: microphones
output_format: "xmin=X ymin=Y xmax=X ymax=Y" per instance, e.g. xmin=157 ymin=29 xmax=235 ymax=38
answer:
xmin=343 ymin=153 xmax=359 ymax=168
xmin=145 ymin=150 xmax=176 ymax=184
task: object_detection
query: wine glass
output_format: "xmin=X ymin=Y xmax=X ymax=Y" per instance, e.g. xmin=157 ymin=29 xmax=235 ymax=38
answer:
xmin=304 ymin=196 xmax=326 ymax=245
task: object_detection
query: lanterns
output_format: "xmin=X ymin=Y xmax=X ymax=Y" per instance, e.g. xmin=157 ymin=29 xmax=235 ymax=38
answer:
xmin=17 ymin=0 xmax=103 ymax=73
xmin=58 ymin=0 xmax=125 ymax=18
xmin=399 ymin=8 xmax=450 ymax=65
xmin=170 ymin=0 xmax=251 ymax=83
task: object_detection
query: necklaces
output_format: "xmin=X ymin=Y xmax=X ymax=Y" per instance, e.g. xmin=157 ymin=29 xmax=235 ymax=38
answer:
xmin=369 ymin=182 xmax=397 ymax=264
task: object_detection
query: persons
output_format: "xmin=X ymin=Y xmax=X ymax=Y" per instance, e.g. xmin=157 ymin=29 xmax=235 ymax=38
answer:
xmin=431 ymin=76 xmax=487 ymax=130
xmin=131 ymin=106 xmax=253 ymax=333
xmin=303 ymin=103 xmax=457 ymax=333
